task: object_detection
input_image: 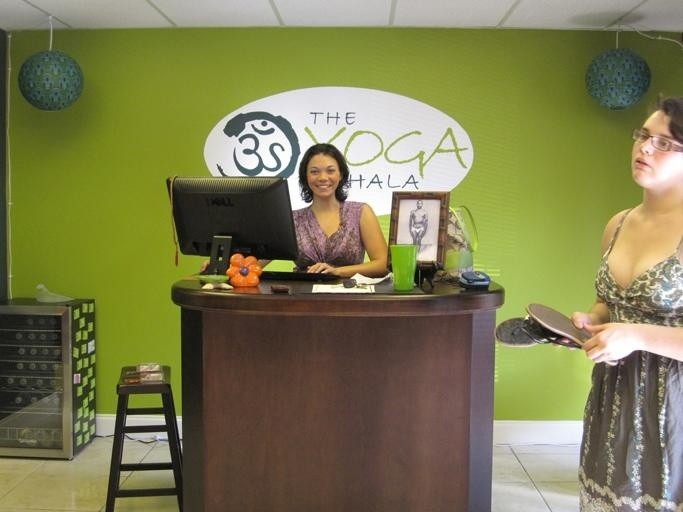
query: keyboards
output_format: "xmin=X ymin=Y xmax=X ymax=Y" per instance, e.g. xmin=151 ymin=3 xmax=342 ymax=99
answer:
xmin=261 ymin=269 xmax=336 ymax=281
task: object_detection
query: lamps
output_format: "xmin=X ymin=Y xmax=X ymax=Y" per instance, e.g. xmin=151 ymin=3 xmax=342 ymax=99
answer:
xmin=16 ymin=16 xmax=85 ymax=113
xmin=583 ymin=17 xmax=652 ymax=110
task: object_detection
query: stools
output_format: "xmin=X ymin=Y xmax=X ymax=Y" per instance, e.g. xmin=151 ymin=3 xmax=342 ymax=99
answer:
xmin=105 ymin=361 xmax=182 ymax=512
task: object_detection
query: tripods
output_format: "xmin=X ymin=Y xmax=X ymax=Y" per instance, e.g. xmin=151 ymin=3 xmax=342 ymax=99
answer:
xmin=166 ymin=176 xmax=298 ymax=274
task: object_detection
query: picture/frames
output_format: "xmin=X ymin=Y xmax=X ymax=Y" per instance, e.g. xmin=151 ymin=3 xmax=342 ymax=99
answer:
xmin=385 ymin=190 xmax=450 ymax=273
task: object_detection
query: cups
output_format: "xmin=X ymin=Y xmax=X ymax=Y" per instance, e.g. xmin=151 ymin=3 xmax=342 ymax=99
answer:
xmin=390 ymin=245 xmax=417 ymax=290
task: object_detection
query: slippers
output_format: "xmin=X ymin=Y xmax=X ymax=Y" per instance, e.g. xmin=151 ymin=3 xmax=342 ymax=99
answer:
xmin=521 ymin=302 xmax=620 ymax=367
xmin=492 ymin=316 xmax=574 ymax=348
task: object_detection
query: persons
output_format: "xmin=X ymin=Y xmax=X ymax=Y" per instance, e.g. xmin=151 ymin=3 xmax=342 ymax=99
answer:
xmin=198 ymin=143 xmax=390 ymax=278
xmin=407 ymin=199 xmax=428 ymax=252
xmin=567 ymin=99 xmax=682 ymax=512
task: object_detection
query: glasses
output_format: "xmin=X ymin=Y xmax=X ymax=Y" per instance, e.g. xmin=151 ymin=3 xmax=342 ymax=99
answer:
xmin=631 ymin=127 xmax=682 ymax=153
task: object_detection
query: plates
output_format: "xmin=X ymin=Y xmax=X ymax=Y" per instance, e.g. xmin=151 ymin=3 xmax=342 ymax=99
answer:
xmin=196 ymin=274 xmax=230 ymax=283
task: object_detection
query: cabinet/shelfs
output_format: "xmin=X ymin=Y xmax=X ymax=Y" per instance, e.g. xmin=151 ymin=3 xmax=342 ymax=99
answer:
xmin=0 ymin=295 xmax=99 ymax=461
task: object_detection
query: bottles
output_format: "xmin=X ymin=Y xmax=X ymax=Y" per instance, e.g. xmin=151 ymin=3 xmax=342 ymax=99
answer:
xmin=7 ymin=315 xmax=62 ymax=406
